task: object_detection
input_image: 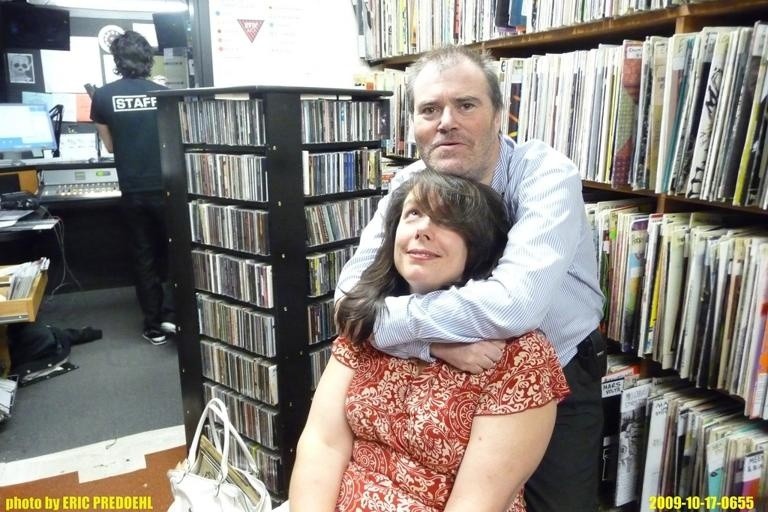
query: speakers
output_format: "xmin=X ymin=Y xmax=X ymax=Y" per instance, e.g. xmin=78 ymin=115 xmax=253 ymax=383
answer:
xmin=0 ymin=3 xmax=70 ymax=51
xmin=152 ymin=12 xmax=186 ymax=51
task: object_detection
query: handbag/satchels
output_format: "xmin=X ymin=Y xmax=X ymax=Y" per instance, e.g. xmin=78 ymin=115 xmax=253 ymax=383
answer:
xmin=166 ymin=397 xmax=272 ymax=512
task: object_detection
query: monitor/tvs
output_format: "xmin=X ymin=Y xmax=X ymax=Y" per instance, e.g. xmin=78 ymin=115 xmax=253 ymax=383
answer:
xmin=1 ymin=103 xmax=57 ymax=160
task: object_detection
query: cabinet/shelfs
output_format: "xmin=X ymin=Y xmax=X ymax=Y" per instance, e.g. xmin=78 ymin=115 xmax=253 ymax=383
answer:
xmin=0 ymin=261 xmax=49 ymax=379
xmin=348 ymin=1 xmax=768 ymax=512
xmin=147 ymin=85 xmax=394 ymax=505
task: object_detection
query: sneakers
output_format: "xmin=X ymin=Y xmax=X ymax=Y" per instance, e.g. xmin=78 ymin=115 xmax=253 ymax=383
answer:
xmin=160 ymin=317 xmax=181 ymax=335
xmin=142 ymin=328 xmax=167 ymax=346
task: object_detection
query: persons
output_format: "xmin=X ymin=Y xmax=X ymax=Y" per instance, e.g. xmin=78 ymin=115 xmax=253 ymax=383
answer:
xmin=87 ymin=32 xmax=183 ymax=348
xmin=331 ymin=42 xmax=605 ymax=512
xmin=284 ymin=168 xmax=571 ymax=512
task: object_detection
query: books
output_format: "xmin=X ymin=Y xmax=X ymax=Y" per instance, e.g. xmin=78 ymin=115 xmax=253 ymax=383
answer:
xmin=0 ymin=258 xmax=50 ymax=421
xmin=172 ymin=1 xmax=765 ymax=512
